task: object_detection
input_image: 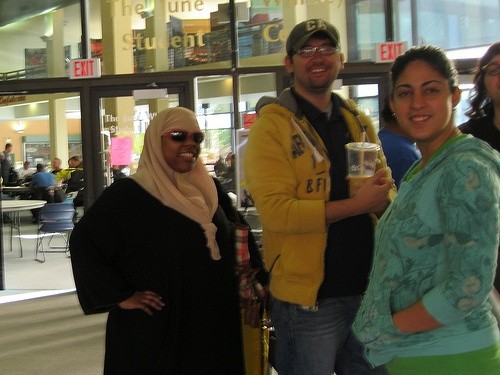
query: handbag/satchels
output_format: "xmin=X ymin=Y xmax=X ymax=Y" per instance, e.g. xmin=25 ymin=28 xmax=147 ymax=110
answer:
xmin=235 ymin=224 xmax=267 ymax=329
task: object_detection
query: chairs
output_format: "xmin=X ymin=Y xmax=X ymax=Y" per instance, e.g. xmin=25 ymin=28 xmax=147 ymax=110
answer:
xmin=35 ymin=203 xmax=76 ymax=263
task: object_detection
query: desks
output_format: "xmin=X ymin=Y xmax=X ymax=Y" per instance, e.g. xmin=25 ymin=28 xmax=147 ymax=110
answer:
xmin=0 ymin=199 xmax=48 ymax=258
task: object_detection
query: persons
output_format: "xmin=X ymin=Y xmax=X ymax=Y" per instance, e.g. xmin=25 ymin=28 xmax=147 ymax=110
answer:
xmin=350 ymin=45 xmax=500 ymax=375
xmin=69 ymin=107 xmax=270 ymax=375
xmin=377 ymin=96 xmax=423 ymax=194
xmin=214 ymin=151 xmax=237 ymax=188
xmin=0 ymin=143 xmax=84 ymax=223
xmin=456 ymin=40 xmax=500 ymax=152
xmin=244 ymin=17 xmax=399 ymax=375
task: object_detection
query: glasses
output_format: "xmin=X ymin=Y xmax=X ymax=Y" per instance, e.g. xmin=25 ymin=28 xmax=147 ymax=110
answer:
xmin=295 ymin=45 xmax=337 ymax=57
xmin=161 ymin=129 xmax=204 ymax=143
xmin=480 ymin=61 xmax=500 ymax=77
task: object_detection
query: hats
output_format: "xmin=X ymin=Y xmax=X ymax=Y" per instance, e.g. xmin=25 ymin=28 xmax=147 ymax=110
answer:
xmin=286 ymin=19 xmax=341 ymax=59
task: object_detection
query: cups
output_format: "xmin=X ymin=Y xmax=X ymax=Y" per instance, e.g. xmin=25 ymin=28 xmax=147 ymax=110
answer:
xmin=345 ymin=142 xmax=380 ymax=199
